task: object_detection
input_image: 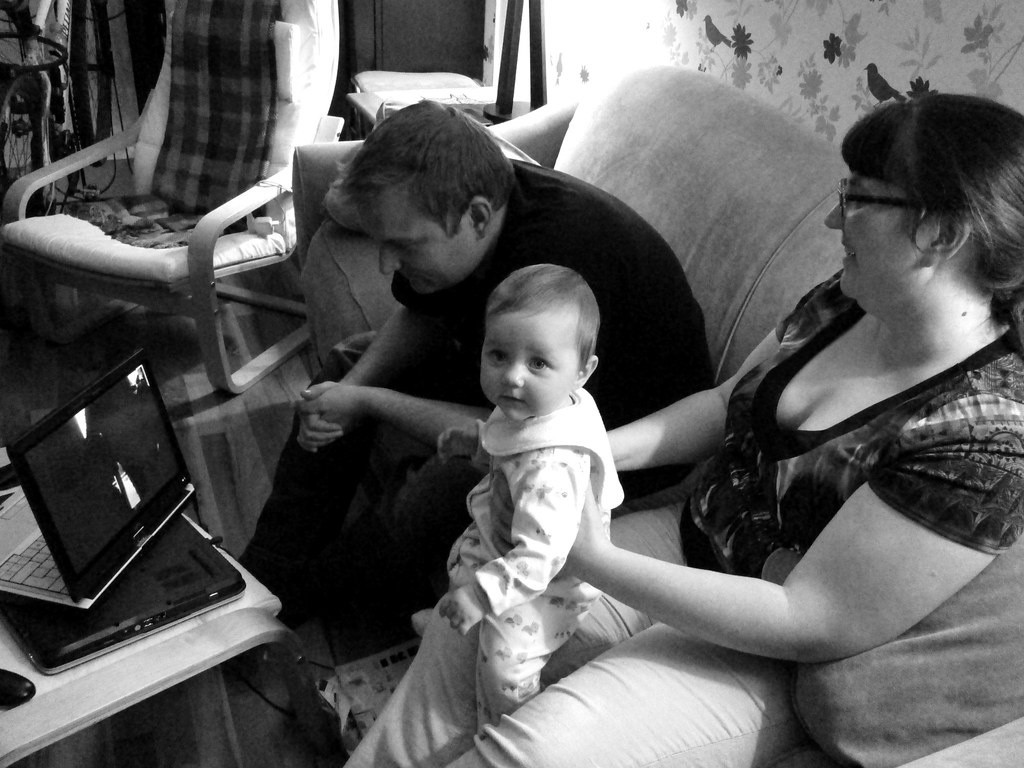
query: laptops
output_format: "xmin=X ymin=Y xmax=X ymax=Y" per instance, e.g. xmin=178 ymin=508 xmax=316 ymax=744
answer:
xmin=0 ymin=347 xmax=247 ymax=677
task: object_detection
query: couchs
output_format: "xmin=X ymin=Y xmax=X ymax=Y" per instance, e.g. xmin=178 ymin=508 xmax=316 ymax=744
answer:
xmin=290 ymin=67 xmax=1024 ymax=768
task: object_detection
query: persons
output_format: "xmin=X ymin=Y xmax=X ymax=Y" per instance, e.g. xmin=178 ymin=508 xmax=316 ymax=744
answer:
xmin=234 ymin=99 xmax=716 ymax=666
xmin=342 ymin=93 xmax=1024 ymax=768
xmin=437 ymin=263 xmax=626 ymax=738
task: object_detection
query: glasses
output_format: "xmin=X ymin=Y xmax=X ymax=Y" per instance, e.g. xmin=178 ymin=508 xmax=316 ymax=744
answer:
xmin=837 ymin=176 xmax=935 ymax=219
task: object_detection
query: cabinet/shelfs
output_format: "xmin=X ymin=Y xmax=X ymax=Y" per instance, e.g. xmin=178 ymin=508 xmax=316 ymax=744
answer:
xmin=344 ymin=87 xmax=558 ymax=139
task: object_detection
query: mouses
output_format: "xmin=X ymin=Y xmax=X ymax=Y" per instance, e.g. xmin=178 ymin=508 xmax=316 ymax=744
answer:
xmin=0 ymin=670 xmax=35 ymax=708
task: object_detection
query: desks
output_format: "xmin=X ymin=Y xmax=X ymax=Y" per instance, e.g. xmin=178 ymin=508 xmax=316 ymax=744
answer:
xmin=0 ymin=446 xmax=285 ymax=768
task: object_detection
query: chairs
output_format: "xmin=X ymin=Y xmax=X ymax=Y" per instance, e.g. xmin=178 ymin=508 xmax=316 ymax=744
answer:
xmin=0 ymin=0 xmax=340 ymax=397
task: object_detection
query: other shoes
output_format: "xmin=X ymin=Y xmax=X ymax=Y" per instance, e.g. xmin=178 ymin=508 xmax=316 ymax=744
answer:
xmin=241 ymin=546 xmax=323 ymax=628
